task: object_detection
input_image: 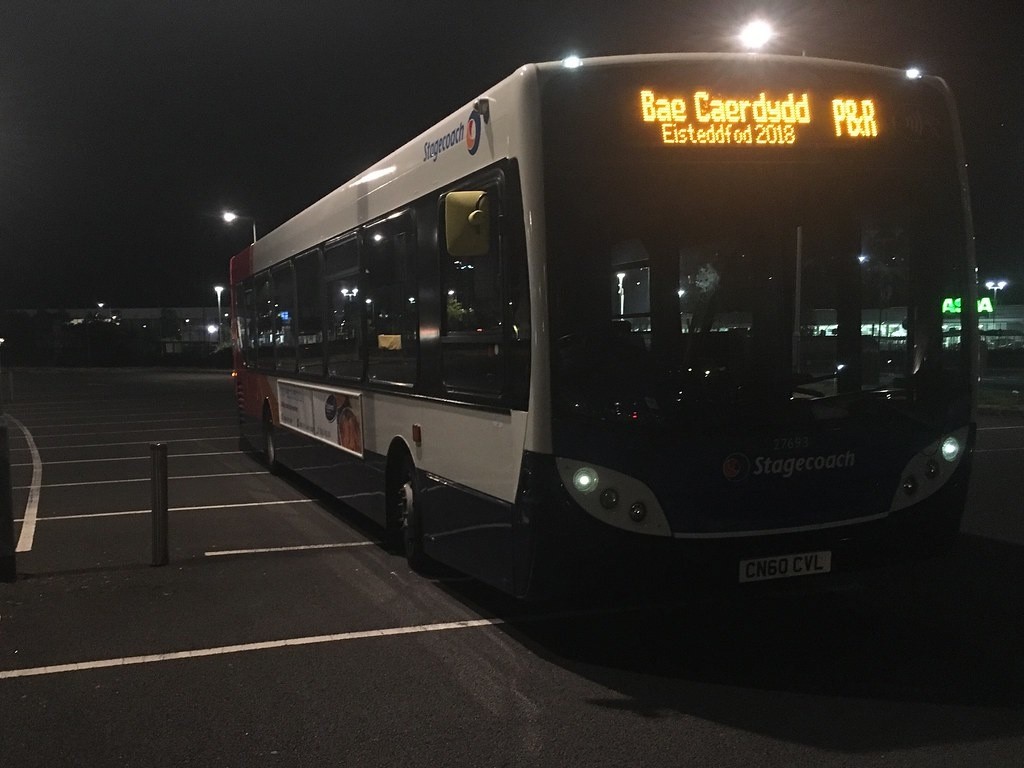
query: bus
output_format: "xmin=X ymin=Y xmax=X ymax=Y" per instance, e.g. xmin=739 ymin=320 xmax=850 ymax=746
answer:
xmin=223 ymin=49 xmax=986 ymax=641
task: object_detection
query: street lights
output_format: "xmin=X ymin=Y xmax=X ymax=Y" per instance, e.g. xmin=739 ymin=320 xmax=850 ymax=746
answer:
xmin=223 ymin=211 xmax=258 ymax=244
xmin=986 ymin=280 xmax=1007 ymax=322
xmin=214 ymin=286 xmax=225 ymax=365
